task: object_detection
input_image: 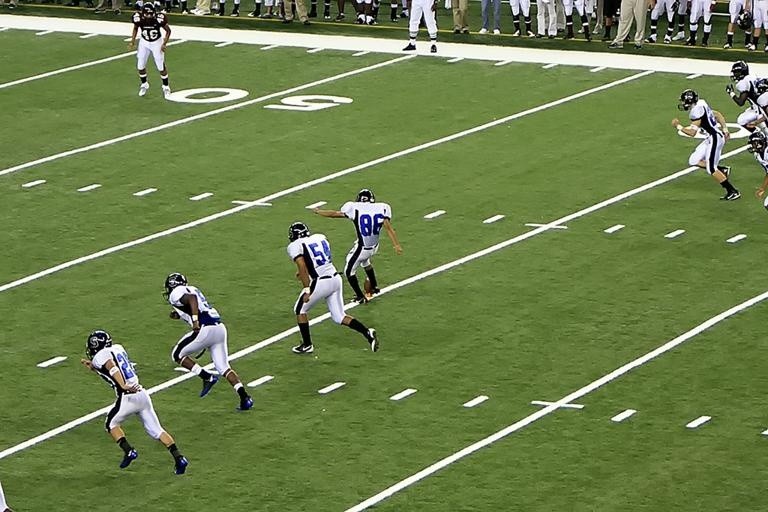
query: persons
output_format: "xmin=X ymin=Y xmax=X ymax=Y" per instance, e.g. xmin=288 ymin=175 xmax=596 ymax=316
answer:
xmin=314 ymin=188 xmax=403 ymax=304
xmin=673 ymin=89 xmax=741 ymax=201
xmin=286 ymin=221 xmax=379 ymax=353
xmin=131 ymin=3 xmax=171 ymax=96
xmin=163 ymin=272 xmax=254 ymax=411
xmin=81 ymin=330 xmax=188 ymax=475
xmin=726 ymin=60 xmax=768 ymax=209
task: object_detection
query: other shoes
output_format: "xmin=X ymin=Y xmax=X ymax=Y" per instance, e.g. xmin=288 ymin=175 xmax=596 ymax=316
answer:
xmin=114 ymin=9 xmax=121 ymax=15
xmin=282 ymin=20 xmax=290 ymax=24
xmin=303 ymin=21 xmax=311 ymax=25
xmin=93 ymin=8 xmax=103 ymax=14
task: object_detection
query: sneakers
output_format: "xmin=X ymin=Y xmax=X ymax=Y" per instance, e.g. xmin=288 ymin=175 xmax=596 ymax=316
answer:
xmin=236 ymin=397 xmax=254 ymax=410
xmin=174 ymin=456 xmax=188 ymax=474
xmin=199 ymin=374 xmax=219 ymax=399
xmin=367 ymin=327 xmax=380 ymax=352
xmin=163 ymin=8 xmax=332 ymax=22
xmin=334 ymin=8 xmax=409 ymax=25
xmin=684 ymin=35 xmax=768 ymax=53
xmin=120 ymin=448 xmax=138 ymax=469
xmin=351 ymin=286 xmax=381 ymax=304
xmin=162 ymin=84 xmax=171 ymax=97
xmin=137 ymin=83 xmax=149 ymax=96
xmin=452 ymin=27 xmax=686 ymax=50
xmin=431 ymin=44 xmax=437 ymax=53
xmin=722 ymin=166 xmax=731 ymax=178
xmin=292 ymin=344 xmax=314 ymax=353
xmin=402 ymin=42 xmax=416 ymax=50
xmin=720 ymin=192 xmax=742 ymax=200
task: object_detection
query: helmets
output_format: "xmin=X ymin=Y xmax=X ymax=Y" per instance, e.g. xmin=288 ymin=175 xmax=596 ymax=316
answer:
xmin=730 ymin=60 xmax=749 ymax=82
xmin=84 ymin=329 xmax=114 ymax=360
xmin=678 ymin=89 xmax=698 ymax=111
xmin=288 ymin=221 xmax=309 ymax=241
xmin=357 ymin=189 xmax=375 ymax=202
xmin=755 ymin=78 xmax=768 ymax=95
xmin=748 ymin=131 xmax=766 ymax=153
xmin=161 ymin=273 xmax=187 ymax=300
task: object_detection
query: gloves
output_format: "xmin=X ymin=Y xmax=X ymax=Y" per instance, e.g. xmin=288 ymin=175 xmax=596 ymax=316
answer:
xmin=725 ymin=84 xmax=735 ymax=98
